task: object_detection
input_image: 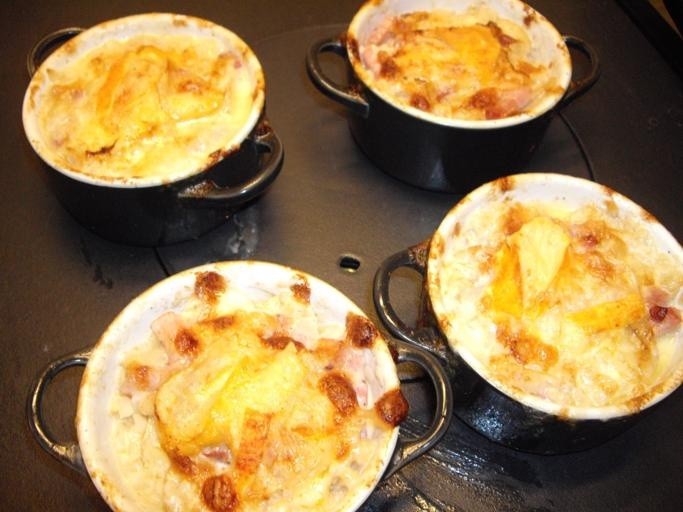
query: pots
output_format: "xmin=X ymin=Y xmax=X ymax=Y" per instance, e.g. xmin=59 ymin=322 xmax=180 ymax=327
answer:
xmin=302 ymin=0 xmax=602 ymax=196
xmin=24 ymin=258 xmax=455 ymax=512
xmin=371 ymin=170 xmax=683 ymax=459
xmin=19 ymin=11 xmax=287 ymax=250
